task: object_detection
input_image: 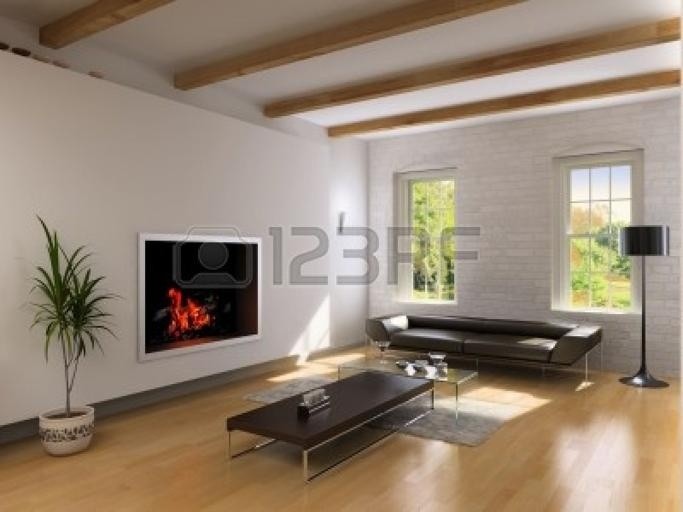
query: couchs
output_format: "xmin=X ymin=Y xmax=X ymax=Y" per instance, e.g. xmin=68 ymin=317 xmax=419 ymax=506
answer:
xmin=365 ymin=314 xmax=602 ymax=387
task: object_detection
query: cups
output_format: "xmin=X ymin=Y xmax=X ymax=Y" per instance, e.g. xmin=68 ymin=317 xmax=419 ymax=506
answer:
xmin=395 ymin=352 xmax=448 ymax=380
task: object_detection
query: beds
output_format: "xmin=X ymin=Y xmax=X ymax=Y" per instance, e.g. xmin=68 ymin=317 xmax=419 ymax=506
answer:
xmin=224 ymin=372 xmax=436 ymax=483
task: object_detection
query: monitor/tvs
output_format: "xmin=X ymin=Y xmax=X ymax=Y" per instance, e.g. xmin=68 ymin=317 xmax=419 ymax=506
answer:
xmin=138 ymin=233 xmax=262 ymax=360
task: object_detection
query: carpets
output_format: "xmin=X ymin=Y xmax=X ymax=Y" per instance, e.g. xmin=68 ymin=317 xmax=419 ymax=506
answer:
xmin=243 ymin=362 xmax=519 ymax=448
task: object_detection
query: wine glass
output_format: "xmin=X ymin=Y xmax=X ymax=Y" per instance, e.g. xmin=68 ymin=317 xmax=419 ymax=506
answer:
xmin=375 ymin=341 xmax=390 ymax=364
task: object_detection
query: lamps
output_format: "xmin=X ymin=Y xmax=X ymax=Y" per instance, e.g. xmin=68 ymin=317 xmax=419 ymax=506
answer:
xmin=617 ymin=225 xmax=670 ymax=389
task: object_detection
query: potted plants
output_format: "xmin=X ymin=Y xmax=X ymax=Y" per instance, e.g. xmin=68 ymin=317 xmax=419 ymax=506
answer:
xmin=19 ymin=213 xmax=126 ymax=457
